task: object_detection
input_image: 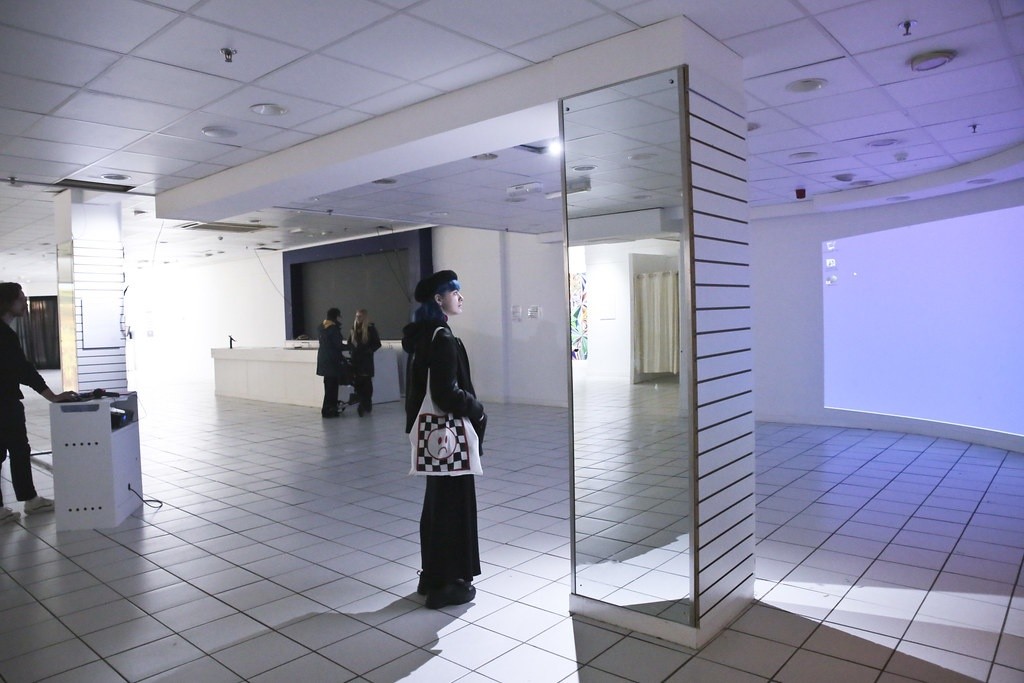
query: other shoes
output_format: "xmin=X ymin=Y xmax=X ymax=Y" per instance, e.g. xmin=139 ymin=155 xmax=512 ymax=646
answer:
xmin=358 ymin=405 xmax=364 ymax=417
xmin=417 ymin=571 xmax=436 ymax=596
xmin=322 ymin=409 xmax=339 ymax=417
xmin=424 ymin=582 xmax=476 ymax=610
xmin=366 ymin=402 xmax=372 ymax=413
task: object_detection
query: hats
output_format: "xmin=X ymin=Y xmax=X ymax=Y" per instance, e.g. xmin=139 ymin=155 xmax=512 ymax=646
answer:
xmin=414 ymin=270 xmax=459 ymax=304
xmin=327 ymin=308 xmax=342 ymax=318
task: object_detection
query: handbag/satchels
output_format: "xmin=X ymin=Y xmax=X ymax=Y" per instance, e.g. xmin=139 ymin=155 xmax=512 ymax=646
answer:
xmin=339 ymin=357 xmax=354 ymax=386
xmin=407 ymin=390 xmax=484 ymax=476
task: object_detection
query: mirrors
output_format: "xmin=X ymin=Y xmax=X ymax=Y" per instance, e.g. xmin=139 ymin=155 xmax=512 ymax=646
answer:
xmin=55 ymin=238 xmax=79 ymax=394
xmin=554 ymin=65 xmax=700 ymax=630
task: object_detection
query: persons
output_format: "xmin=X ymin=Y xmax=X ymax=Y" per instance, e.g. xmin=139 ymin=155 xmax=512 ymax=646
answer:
xmin=401 ymin=270 xmax=488 ymax=610
xmin=0 ymin=282 xmax=78 ymax=525
xmin=315 ymin=308 xmax=382 ymax=418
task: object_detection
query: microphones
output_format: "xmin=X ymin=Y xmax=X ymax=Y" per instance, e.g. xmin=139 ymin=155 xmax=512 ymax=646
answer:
xmin=93 ymin=388 xmax=120 ymax=398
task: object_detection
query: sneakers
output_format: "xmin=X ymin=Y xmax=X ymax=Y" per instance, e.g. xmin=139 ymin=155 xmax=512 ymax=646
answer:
xmin=24 ymin=496 xmax=55 ymax=515
xmin=0 ymin=507 xmax=21 ymax=525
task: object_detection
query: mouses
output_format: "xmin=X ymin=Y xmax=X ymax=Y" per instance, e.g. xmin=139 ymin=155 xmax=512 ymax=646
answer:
xmin=73 ymin=396 xmax=82 ymax=401
